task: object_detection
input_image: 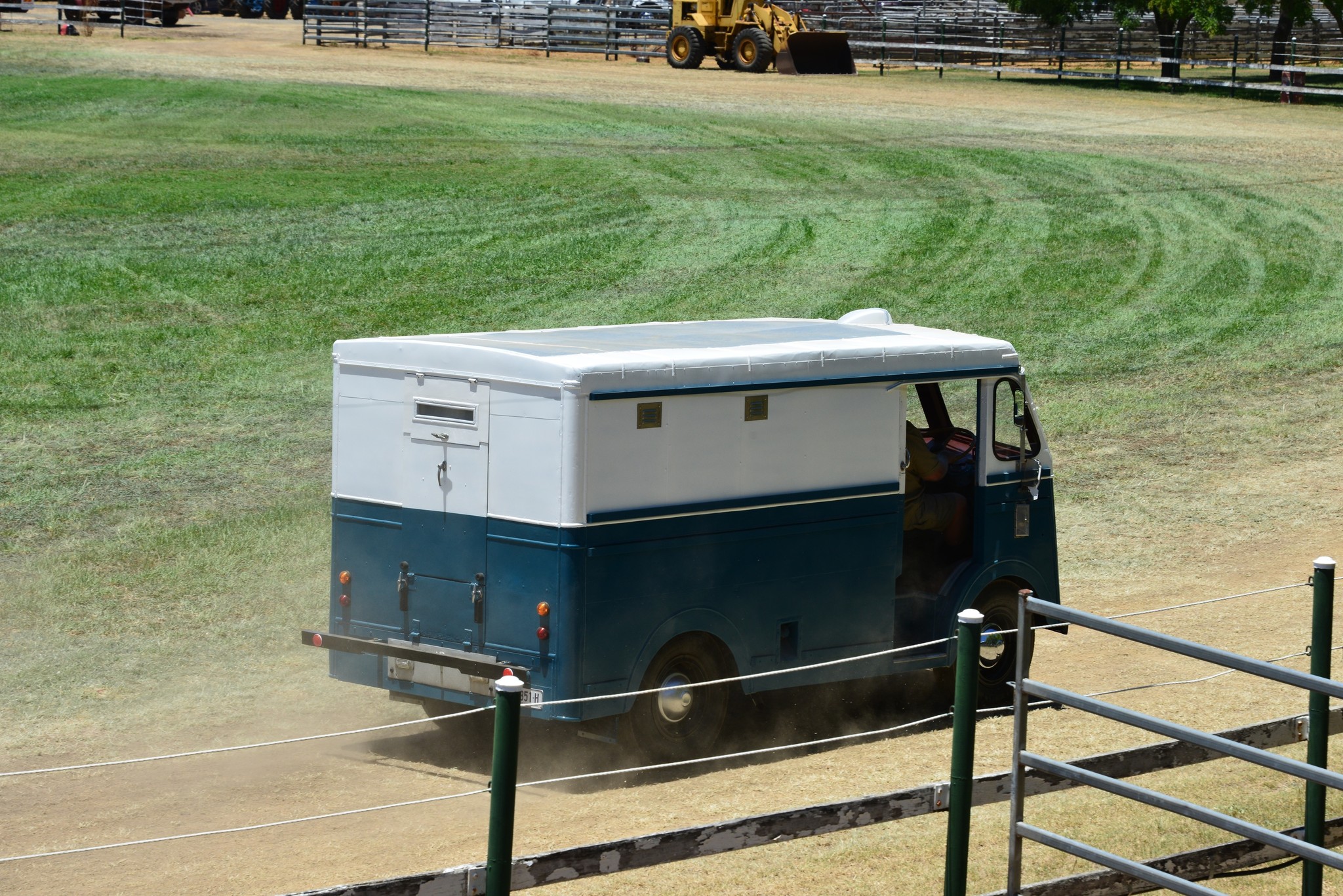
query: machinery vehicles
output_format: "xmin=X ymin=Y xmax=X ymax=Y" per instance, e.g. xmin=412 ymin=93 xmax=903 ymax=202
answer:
xmin=664 ymin=0 xmax=859 ymax=76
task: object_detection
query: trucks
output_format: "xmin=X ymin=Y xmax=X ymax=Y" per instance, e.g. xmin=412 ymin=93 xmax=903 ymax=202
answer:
xmin=299 ymin=307 xmax=1069 ymax=772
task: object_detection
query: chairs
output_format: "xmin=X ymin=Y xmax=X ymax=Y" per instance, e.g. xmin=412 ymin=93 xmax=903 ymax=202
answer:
xmin=903 ymin=530 xmax=943 ymax=562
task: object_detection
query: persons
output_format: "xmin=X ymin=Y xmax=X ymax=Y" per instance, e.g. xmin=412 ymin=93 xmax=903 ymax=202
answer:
xmin=904 ymin=420 xmax=967 ymax=561
